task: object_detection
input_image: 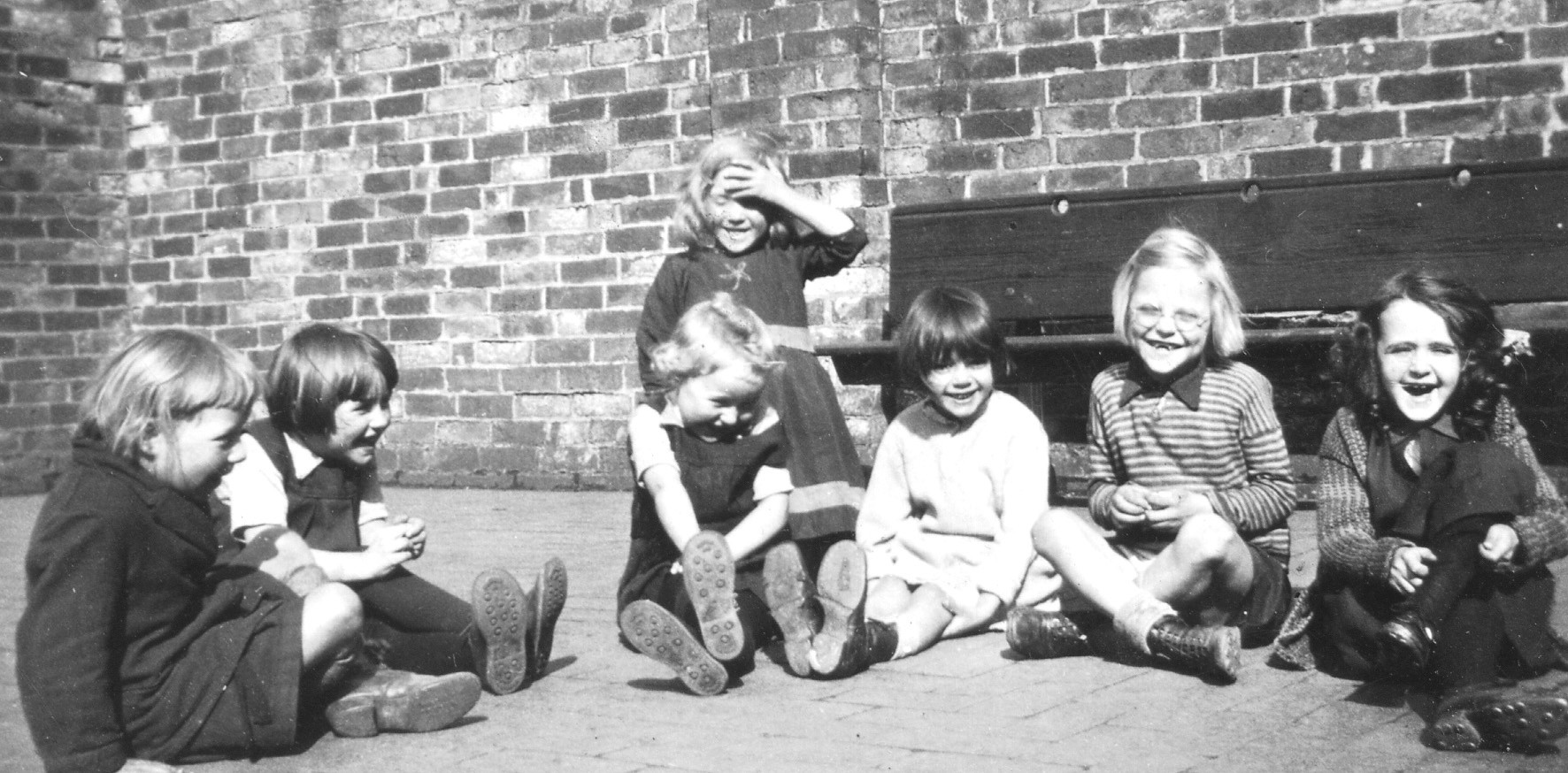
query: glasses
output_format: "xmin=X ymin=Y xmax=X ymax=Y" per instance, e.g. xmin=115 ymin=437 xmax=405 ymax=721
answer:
xmin=1124 ymin=304 xmax=1219 ymax=334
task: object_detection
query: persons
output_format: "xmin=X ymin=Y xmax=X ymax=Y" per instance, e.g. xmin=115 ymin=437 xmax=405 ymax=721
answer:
xmin=634 ymin=129 xmax=869 ymax=543
xmin=1271 ymin=270 xmax=1567 ymax=753
xmin=14 ymin=324 xmax=479 ymax=773
xmin=1004 ymin=231 xmax=1300 ymax=684
xmin=762 ymin=285 xmax=1077 ymax=680
xmin=616 ymin=293 xmax=797 ymax=699
xmin=215 ymin=323 xmax=568 ymax=697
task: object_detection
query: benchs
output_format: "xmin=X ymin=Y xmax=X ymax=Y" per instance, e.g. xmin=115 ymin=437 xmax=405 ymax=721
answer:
xmin=808 ymin=153 xmax=1568 ymax=510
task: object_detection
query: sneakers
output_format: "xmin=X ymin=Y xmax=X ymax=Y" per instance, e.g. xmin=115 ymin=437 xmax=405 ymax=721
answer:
xmin=1433 ymin=686 xmax=1568 ymax=752
xmin=1006 ymin=608 xmax=1106 ymax=659
xmin=1147 ymin=617 xmax=1239 ymax=682
xmin=1376 ymin=612 xmax=1423 ymax=675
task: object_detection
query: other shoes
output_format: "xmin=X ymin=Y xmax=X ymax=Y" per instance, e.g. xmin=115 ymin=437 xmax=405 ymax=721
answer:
xmin=622 ymin=602 xmax=731 ymax=696
xmin=471 ymin=571 xmax=529 ymax=696
xmin=682 ymin=529 xmax=745 ymax=664
xmin=521 ymin=557 xmax=567 ymax=680
xmin=323 ymin=659 xmax=481 ymax=738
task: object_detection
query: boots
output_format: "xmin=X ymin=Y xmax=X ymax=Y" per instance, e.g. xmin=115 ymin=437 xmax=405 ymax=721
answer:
xmin=809 ymin=538 xmax=896 ymax=678
xmin=764 ymin=539 xmax=817 ymax=680
